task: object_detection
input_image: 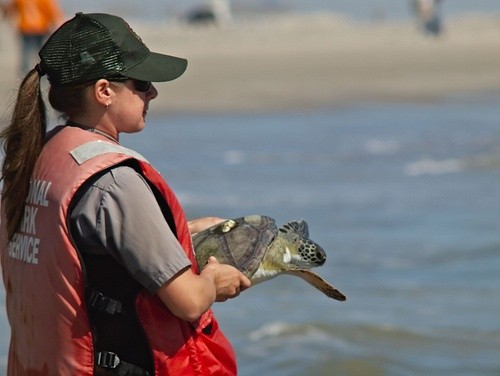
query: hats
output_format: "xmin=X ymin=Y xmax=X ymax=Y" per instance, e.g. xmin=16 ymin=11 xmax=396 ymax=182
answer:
xmin=39 ymin=12 xmax=188 ymax=82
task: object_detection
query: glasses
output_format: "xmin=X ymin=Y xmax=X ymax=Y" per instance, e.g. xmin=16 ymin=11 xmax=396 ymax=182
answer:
xmin=104 ymin=77 xmax=152 ymax=92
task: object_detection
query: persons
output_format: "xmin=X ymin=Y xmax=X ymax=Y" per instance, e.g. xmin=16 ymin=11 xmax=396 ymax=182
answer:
xmin=0 ymin=14 xmax=252 ymax=375
xmin=7 ymin=0 xmax=63 ymax=75
